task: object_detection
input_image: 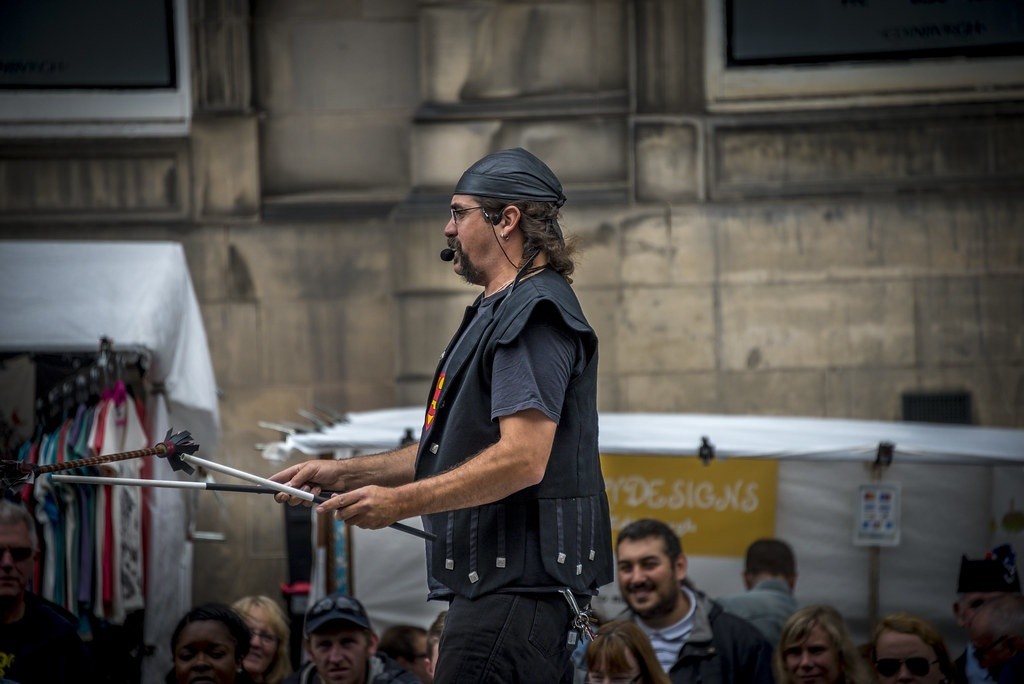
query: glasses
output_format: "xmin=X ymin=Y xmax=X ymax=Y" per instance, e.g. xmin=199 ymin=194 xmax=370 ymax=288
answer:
xmin=973 ymin=635 xmax=1008 ymax=664
xmin=0 ymin=543 xmax=35 ymax=563
xmin=305 ymin=596 xmax=367 ymax=623
xmin=874 ymin=655 xmax=939 ymax=679
xmin=965 ymin=597 xmax=986 ymax=609
xmin=450 ymin=205 xmax=486 ymax=224
xmin=248 ymin=627 xmax=280 ymax=644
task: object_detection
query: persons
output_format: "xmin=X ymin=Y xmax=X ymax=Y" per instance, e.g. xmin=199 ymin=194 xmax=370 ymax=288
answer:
xmin=617 ymin=519 xmax=1024 ymax=684
xmin=0 ymin=498 xmax=93 ymax=684
xmin=166 ymin=591 xmax=449 ymax=684
xmin=577 ymin=621 xmax=672 ymax=684
xmin=267 ymin=147 xmax=614 ymax=684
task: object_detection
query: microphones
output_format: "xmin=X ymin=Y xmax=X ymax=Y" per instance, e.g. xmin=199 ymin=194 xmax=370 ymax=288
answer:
xmin=441 ymin=249 xmax=456 ymax=261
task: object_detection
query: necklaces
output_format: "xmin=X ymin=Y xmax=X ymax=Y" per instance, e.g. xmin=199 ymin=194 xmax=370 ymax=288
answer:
xmin=483 ymin=277 xmax=515 ymax=299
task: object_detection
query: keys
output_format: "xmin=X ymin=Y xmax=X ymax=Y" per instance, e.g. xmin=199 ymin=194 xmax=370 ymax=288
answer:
xmin=571 ymin=603 xmax=599 ymax=643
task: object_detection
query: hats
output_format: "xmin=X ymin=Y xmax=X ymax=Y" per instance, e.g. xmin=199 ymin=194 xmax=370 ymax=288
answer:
xmin=305 ymin=594 xmax=371 ymax=636
xmin=957 ymin=543 xmax=1021 ymax=594
